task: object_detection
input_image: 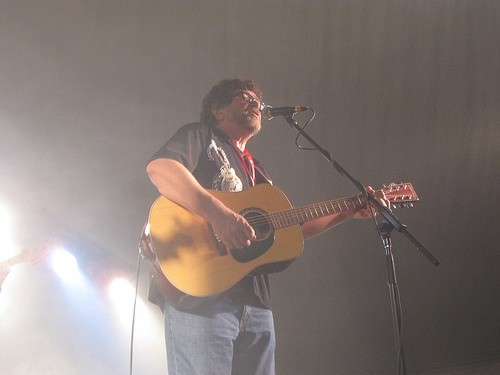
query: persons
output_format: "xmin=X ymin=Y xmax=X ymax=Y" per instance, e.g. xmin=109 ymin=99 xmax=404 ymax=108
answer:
xmin=146 ymin=80 xmax=391 ymax=375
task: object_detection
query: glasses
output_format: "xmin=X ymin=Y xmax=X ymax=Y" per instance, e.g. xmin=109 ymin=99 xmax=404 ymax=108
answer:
xmin=234 ymin=91 xmax=264 ymax=111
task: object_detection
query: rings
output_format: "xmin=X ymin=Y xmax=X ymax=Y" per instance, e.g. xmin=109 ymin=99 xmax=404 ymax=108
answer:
xmin=374 ymin=212 xmax=378 ymax=216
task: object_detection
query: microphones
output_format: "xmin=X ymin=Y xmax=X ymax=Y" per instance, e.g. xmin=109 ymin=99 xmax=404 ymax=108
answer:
xmin=262 ymin=105 xmax=309 ymax=120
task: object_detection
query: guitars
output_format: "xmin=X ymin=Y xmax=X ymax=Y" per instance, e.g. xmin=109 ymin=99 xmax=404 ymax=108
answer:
xmin=138 ymin=182 xmax=419 ymax=313
